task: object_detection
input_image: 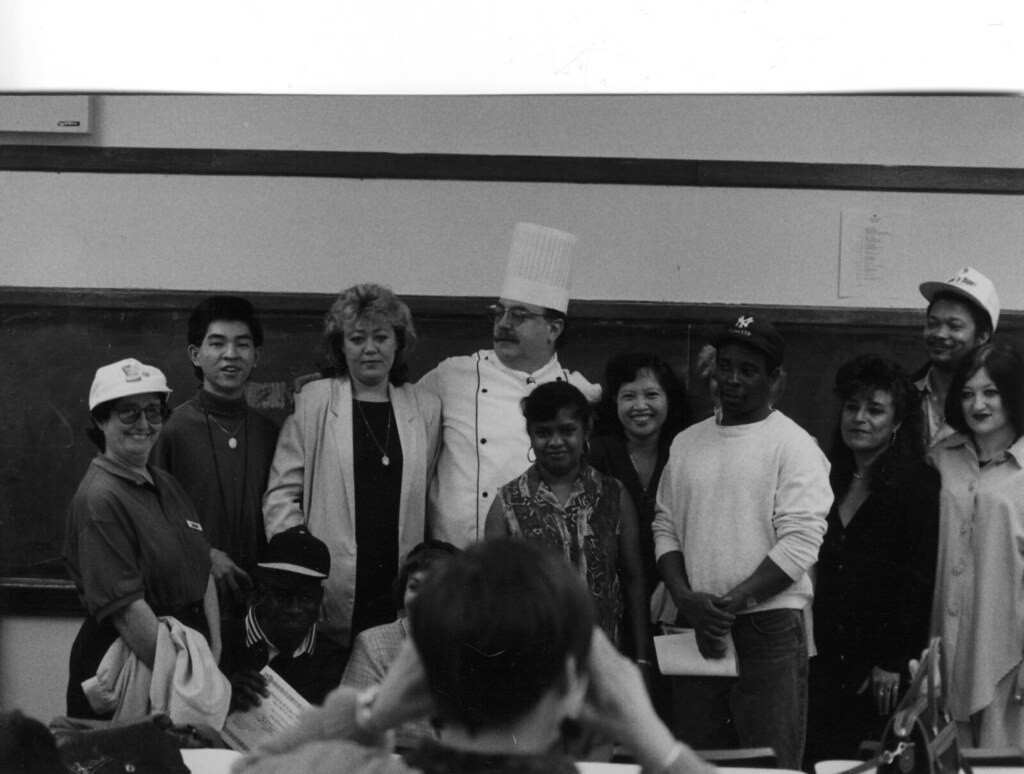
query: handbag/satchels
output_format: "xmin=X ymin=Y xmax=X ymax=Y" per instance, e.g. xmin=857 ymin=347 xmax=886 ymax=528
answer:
xmin=841 ymin=637 xmax=973 ymax=773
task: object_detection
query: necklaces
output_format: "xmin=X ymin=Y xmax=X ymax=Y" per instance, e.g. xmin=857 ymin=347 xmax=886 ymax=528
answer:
xmin=192 ymin=398 xmax=248 ymax=450
xmin=357 ymin=394 xmax=394 ymax=466
xmin=851 ymin=469 xmax=866 ymax=480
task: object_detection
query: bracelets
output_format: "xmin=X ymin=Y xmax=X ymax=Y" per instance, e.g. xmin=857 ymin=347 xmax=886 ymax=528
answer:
xmin=649 ymin=741 xmax=688 ymax=774
xmin=636 ymin=659 xmax=653 ymax=666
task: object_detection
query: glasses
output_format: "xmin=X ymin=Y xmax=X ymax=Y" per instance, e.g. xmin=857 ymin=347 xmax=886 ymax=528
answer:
xmin=486 ymin=303 xmax=555 ymax=326
xmin=108 ymin=401 xmax=174 ymax=424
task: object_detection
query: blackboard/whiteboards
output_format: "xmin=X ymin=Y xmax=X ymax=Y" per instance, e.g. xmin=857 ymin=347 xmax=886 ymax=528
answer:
xmin=0 ymin=284 xmax=1024 ymax=605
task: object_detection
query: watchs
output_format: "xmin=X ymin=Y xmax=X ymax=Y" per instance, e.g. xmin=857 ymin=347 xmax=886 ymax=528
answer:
xmin=735 ymin=584 xmax=758 ymax=610
xmin=355 ymin=686 xmax=377 ymax=731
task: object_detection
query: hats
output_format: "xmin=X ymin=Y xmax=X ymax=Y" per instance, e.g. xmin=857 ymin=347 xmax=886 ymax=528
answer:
xmin=89 ymin=357 xmax=174 ymax=409
xmin=502 ymin=221 xmax=576 ymax=316
xmin=258 ymin=523 xmax=331 ymax=579
xmin=920 ymin=266 xmax=1001 ymax=335
xmin=710 ymin=315 xmax=786 ymax=366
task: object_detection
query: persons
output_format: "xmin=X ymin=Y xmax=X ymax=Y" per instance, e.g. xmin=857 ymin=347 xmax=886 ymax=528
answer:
xmin=482 ymin=381 xmax=649 ymax=761
xmin=234 ymin=537 xmax=719 ymax=774
xmin=66 ymin=358 xmax=222 ymax=719
xmin=652 ymin=268 xmax=1024 ymax=774
xmin=222 ymin=523 xmax=356 ymax=706
xmin=323 ymin=540 xmax=468 ymax=696
xmin=147 ymin=221 xmax=701 ymax=537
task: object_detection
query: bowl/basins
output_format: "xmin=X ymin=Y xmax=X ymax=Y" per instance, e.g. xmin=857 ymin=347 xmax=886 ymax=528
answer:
xmin=180 ymin=748 xmax=239 ymax=774
